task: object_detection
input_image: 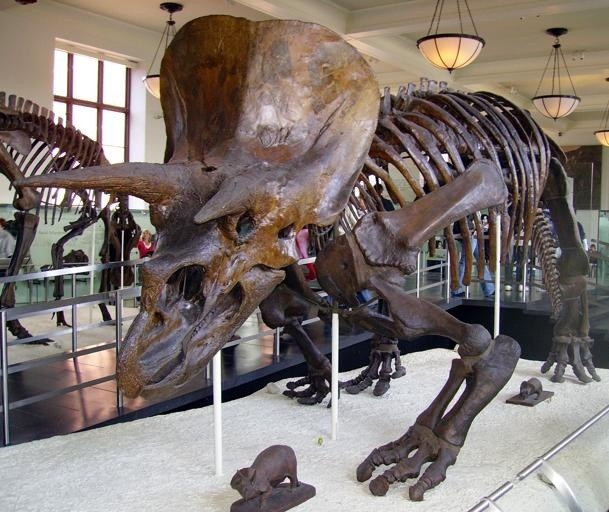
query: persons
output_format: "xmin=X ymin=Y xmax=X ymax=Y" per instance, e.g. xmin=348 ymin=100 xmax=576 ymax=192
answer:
xmin=374 ymin=184 xmax=395 ymax=211
xmin=447 ymin=207 xmax=597 ymax=299
xmin=135 ymin=229 xmax=156 ymax=285
xmin=0 ymin=217 xmax=15 ymax=258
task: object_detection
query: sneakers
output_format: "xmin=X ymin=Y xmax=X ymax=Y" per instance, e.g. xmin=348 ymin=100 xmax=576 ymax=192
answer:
xmin=505 ymin=285 xmax=512 ymax=291
xmin=518 ymin=284 xmax=529 ymax=291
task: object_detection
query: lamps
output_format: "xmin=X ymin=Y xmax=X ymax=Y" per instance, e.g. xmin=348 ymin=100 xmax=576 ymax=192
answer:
xmin=591 ymin=124 xmax=609 ymax=148
xmin=528 ymin=26 xmax=586 ymax=120
xmin=136 ymin=0 xmax=188 ymax=97
xmin=411 ymin=1 xmax=487 ymax=73
